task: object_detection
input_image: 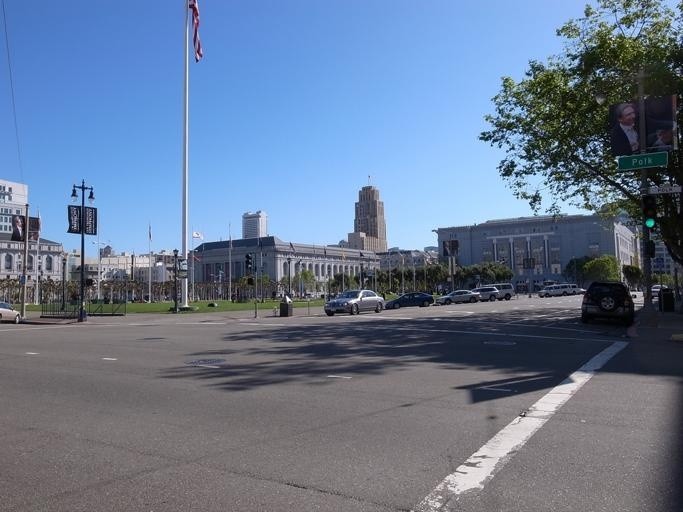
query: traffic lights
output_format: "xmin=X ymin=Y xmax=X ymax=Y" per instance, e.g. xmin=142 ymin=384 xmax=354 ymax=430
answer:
xmin=643 ymin=199 xmax=658 ymax=229
xmin=245 ymin=253 xmax=252 ymax=269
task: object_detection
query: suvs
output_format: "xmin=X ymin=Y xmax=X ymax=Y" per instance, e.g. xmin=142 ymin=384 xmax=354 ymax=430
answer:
xmin=582 ymin=280 xmax=636 ymax=326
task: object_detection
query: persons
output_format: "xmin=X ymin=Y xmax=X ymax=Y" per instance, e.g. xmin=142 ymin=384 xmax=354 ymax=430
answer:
xmin=612 ymin=103 xmax=638 ymax=156
xmin=11 ymin=216 xmax=25 ymax=240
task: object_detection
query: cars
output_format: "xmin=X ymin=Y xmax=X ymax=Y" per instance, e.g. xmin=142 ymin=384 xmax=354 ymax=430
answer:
xmin=0 ymin=301 xmax=22 ymax=324
xmin=324 ymin=289 xmax=384 ymax=316
xmin=537 ymin=283 xmax=587 ymax=298
xmin=651 ymin=285 xmax=668 ymax=300
xmin=436 ymin=283 xmax=516 ymax=305
xmin=385 ymin=292 xmax=434 ymax=309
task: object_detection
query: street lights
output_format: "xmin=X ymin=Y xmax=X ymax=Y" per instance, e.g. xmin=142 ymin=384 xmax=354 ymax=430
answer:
xmin=173 ymin=248 xmax=179 ymax=314
xmin=287 ymin=257 xmax=291 ymax=294
xmin=71 ymin=178 xmax=95 ymax=323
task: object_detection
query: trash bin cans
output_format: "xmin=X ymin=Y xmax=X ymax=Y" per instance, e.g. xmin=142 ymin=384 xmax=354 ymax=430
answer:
xmin=658 ymin=288 xmax=675 ymax=312
xmin=280 ymin=295 xmax=293 ymax=317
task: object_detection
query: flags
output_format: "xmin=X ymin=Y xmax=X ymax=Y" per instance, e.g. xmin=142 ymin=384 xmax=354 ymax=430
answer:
xmin=193 ymin=232 xmax=203 ymax=240
xmin=188 ymin=0 xmax=204 ymax=63
